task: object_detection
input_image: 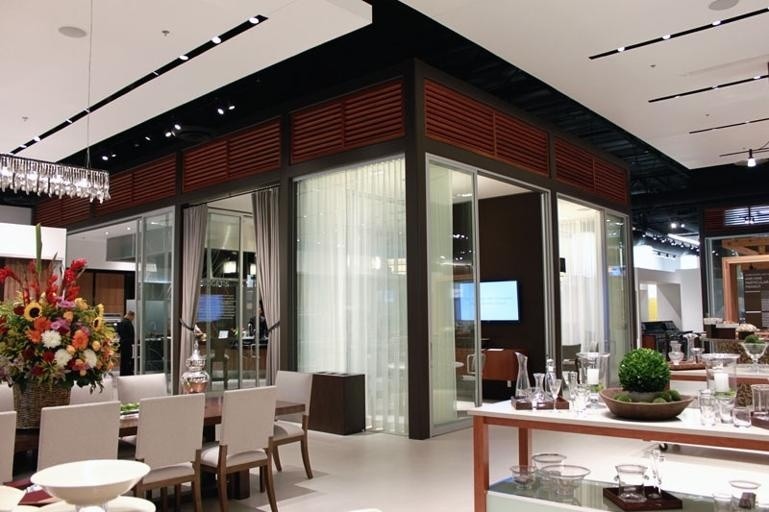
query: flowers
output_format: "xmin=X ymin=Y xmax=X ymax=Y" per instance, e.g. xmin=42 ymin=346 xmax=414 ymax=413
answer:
xmin=0 ymin=222 xmax=119 ymax=392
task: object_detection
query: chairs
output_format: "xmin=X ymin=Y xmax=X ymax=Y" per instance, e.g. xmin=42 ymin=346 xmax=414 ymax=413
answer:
xmin=118 ymin=373 xmax=167 ymax=409
xmin=196 ymin=386 xmax=278 ymax=512
xmin=262 ymin=371 xmax=314 ymax=478
xmin=70 ymin=376 xmax=113 ymax=408
xmin=36 ymin=402 xmax=118 ymax=466
xmin=0 ymin=412 xmax=14 ymax=484
xmin=132 ymin=393 xmax=204 ymax=512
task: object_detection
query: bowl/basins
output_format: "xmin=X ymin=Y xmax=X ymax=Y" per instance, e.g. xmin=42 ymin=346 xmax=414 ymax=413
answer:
xmin=29 ymin=457 xmax=151 ymax=504
xmin=509 ymin=465 xmax=539 ymax=488
xmin=532 ymin=453 xmax=567 ymax=481
xmin=540 ymin=465 xmax=591 ymax=495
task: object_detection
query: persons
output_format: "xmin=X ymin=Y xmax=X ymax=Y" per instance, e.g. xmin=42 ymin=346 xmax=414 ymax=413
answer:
xmin=249 ymin=307 xmax=266 ymax=339
xmin=115 ymin=311 xmax=136 ymax=375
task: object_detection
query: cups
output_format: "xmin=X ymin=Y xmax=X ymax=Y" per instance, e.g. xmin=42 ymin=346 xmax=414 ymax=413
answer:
xmin=750 ymin=384 xmax=768 ymax=417
xmin=561 ymin=358 xmax=576 ymax=388
xmin=700 ymin=353 xmax=741 ymax=399
xmin=567 ymin=371 xmax=578 ymax=411
xmin=615 ymin=464 xmax=648 ymax=503
xmin=548 ymin=379 xmax=562 ymax=413
xmin=738 ymin=342 xmax=769 ymax=369
xmin=697 ymin=387 xmax=718 ymax=427
xmin=715 ymin=396 xmax=736 ymax=424
xmin=575 ymin=352 xmax=611 ymax=412
xmin=728 ymin=480 xmax=762 ymax=512
xmin=731 ymin=407 xmax=751 ymax=427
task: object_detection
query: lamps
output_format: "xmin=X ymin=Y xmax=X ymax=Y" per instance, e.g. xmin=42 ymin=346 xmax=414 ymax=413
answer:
xmin=744 ymin=152 xmax=758 ymax=168
xmin=1 ymin=1 xmax=111 ymax=202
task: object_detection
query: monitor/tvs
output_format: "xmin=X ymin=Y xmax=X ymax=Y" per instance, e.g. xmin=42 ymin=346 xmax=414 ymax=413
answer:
xmin=454 ymin=278 xmax=522 ymax=325
